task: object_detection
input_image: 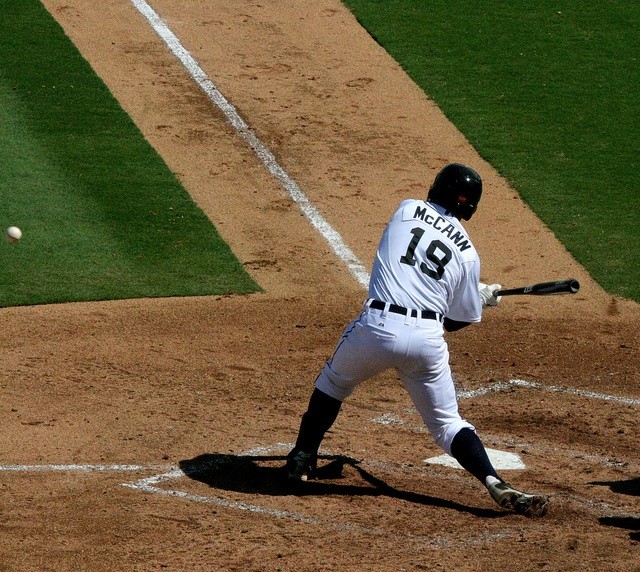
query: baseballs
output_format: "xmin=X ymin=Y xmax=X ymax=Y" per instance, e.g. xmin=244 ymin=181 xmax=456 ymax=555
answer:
xmin=6 ymin=226 xmax=23 ymax=242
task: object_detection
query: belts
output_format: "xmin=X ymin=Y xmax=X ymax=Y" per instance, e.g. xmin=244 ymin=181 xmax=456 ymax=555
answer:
xmin=365 ymin=298 xmax=443 ymax=322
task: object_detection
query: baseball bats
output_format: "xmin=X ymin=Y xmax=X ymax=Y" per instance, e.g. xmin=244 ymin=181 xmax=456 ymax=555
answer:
xmin=494 ymin=280 xmax=581 ymax=296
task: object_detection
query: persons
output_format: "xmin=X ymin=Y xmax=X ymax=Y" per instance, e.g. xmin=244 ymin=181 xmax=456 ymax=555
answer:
xmin=286 ymin=162 xmax=548 ymax=518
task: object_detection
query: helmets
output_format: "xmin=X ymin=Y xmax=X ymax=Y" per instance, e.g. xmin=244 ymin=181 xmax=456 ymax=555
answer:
xmin=426 ymin=162 xmax=482 ymax=221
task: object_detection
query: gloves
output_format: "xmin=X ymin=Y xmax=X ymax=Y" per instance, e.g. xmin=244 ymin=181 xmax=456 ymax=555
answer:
xmin=478 ymin=283 xmax=502 ymax=305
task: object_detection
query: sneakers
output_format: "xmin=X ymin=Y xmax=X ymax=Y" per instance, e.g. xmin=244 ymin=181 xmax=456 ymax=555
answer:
xmin=488 ymin=482 xmax=548 ymax=517
xmin=286 ymin=448 xmax=317 ymax=479
